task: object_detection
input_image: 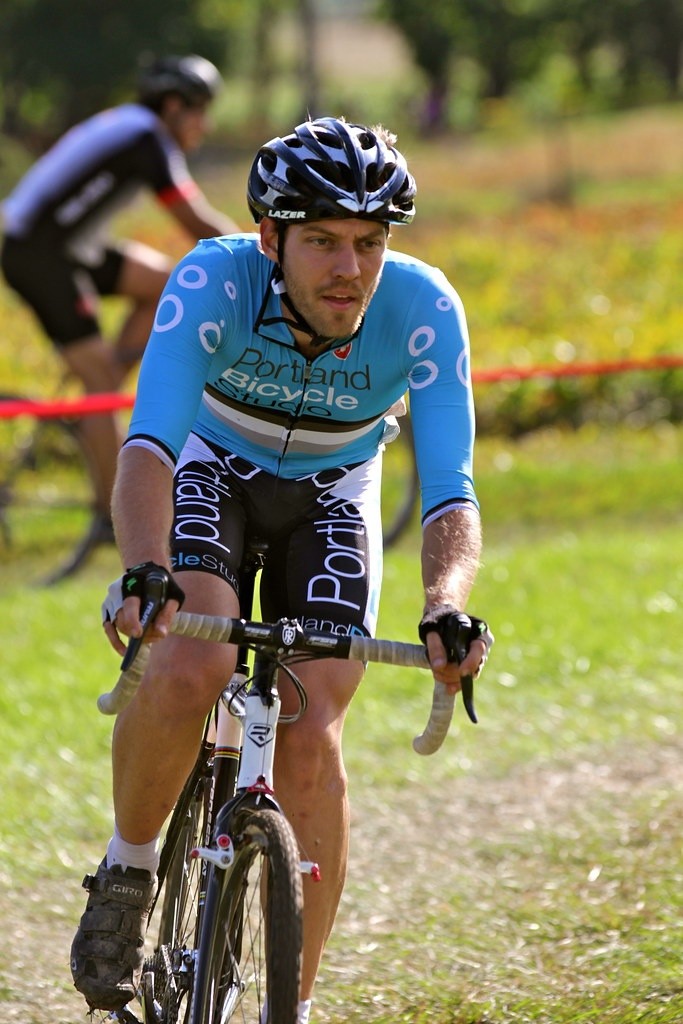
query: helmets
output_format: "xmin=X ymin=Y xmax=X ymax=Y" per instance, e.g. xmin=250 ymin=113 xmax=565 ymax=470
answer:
xmin=138 ymin=52 xmax=218 ymax=106
xmin=244 ymin=116 xmax=416 ymax=229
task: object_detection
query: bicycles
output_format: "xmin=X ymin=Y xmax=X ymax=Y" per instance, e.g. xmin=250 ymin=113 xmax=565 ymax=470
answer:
xmin=0 ymin=356 xmax=114 ymax=590
xmin=84 ymin=560 xmax=486 ymax=1024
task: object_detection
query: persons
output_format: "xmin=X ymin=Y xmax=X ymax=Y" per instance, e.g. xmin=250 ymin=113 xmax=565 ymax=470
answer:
xmin=70 ymin=116 xmax=487 ymax=1021
xmin=0 ymin=55 xmax=250 ymax=533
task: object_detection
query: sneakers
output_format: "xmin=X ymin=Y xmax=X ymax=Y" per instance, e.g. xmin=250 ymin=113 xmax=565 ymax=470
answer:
xmin=69 ymin=856 xmax=160 ymax=1003
